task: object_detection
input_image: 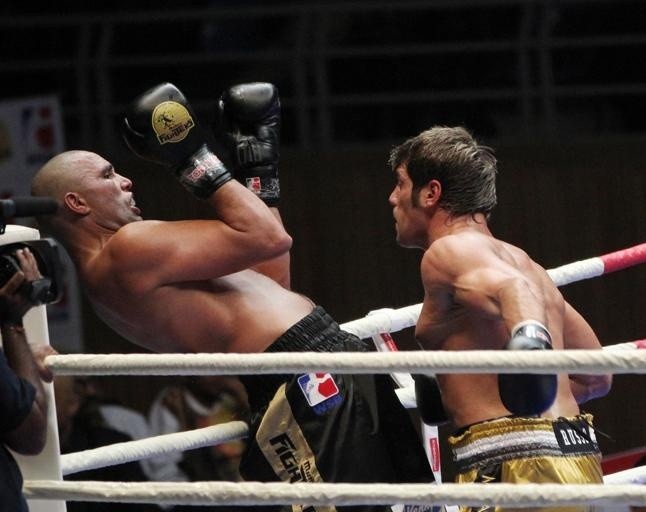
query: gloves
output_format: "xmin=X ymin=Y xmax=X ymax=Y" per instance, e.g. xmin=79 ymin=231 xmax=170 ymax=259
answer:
xmin=412 ymin=372 xmax=446 ymax=426
xmin=496 ymin=320 xmax=559 ymax=417
xmin=119 ymin=79 xmax=233 ymax=200
xmin=209 ymin=81 xmax=285 ymax=208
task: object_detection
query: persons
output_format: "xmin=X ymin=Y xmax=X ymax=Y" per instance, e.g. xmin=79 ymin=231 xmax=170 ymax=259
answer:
xmin=0 ymin=197 xmax=68 ymax=512
xmin=388 ymin=127 xmax=618 ymax=511
xmin=149 ymin=378 xmax=265 ymax=511
xmin=52 ymin=374 xmax=198 ymax=510
xmin=32 ymin=77 xmax=450 ymax=511
